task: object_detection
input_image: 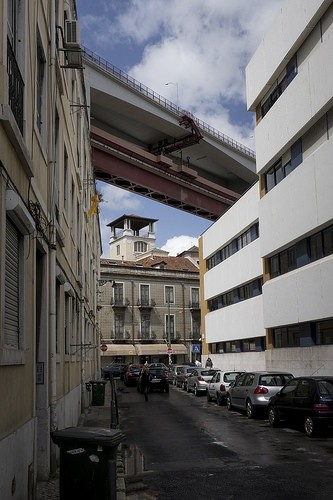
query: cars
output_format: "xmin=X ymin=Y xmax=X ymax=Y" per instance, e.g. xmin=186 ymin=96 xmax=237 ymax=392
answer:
xmin=167 ymin=365 xmax=197 ymax=387
xmin=205 ymin=370 xmax=245 ymax=407
xmin=123 ymin=363 xmax=147 ymax=385
xmin=138 ymin=364 xmax=171 ymax=395
xmin=226 ymin=371 xmax=297 ymax=418
xmin=267 ymin=375 xmax=333 ymax=437
xmin=101 ymin=363 xmax=130 ymax=380
xmin=186 ymin=368 xmax=223 ymax=396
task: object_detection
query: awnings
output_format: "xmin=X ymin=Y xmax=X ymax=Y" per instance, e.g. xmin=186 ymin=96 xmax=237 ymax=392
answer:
xmin=100 ymin=344 xmax=136 ymax=356
xmin=135 ymin=344 xmax=188 ymax=355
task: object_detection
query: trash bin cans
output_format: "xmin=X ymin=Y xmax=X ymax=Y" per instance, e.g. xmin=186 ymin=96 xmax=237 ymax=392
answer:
xmin=49 ymin=423 xmax=127 ymax=500
xmin=89 ymin=380 xmax=108 ymax=407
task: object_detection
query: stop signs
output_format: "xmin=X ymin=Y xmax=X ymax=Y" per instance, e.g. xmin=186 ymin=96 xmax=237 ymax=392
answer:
xmin=100 ymin=345 xmax=107 ymax=351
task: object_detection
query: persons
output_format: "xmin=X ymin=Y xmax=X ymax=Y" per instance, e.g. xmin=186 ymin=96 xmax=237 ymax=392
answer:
xmin=205 ymin=358 xmax=212 ymax=368
xmin=186 ymin=359 xmax=201 ymax=367
